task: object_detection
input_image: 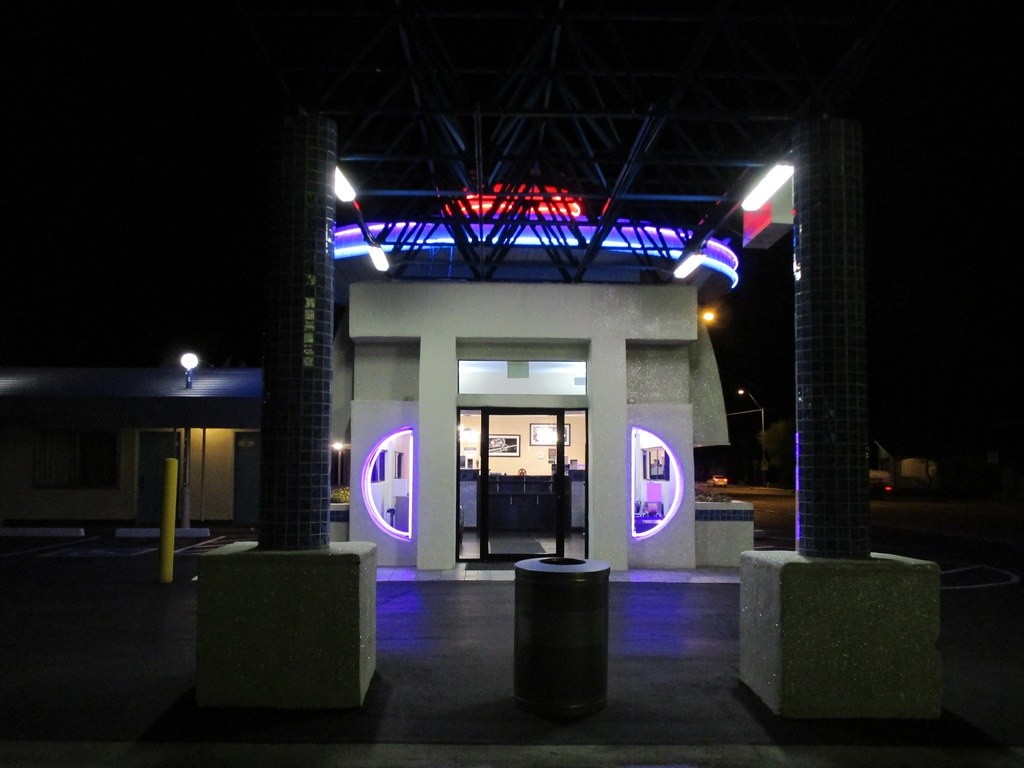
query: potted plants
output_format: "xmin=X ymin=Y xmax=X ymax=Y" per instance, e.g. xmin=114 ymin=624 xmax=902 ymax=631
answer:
xmin=329 ymin=487 xmax=350 ymax=542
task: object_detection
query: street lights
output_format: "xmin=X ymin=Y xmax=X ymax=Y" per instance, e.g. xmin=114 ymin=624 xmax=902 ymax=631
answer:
xmin=737 ymin=389 xmax=768 ymax=487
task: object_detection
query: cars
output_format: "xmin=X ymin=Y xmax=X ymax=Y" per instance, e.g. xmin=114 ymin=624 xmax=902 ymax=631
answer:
xmin=707 ymin=474 xmax=728 ymax=487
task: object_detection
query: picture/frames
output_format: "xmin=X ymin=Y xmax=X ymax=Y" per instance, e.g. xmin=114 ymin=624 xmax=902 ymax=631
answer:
xmin=480 ymin=433 xmax=520 ymax=457
xmin=530 ymin=422 xmax=571 ymax=446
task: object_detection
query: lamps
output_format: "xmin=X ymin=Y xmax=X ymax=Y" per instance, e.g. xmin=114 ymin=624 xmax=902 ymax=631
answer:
xmin=181 ymin=353 xmax=198 ymax=389
